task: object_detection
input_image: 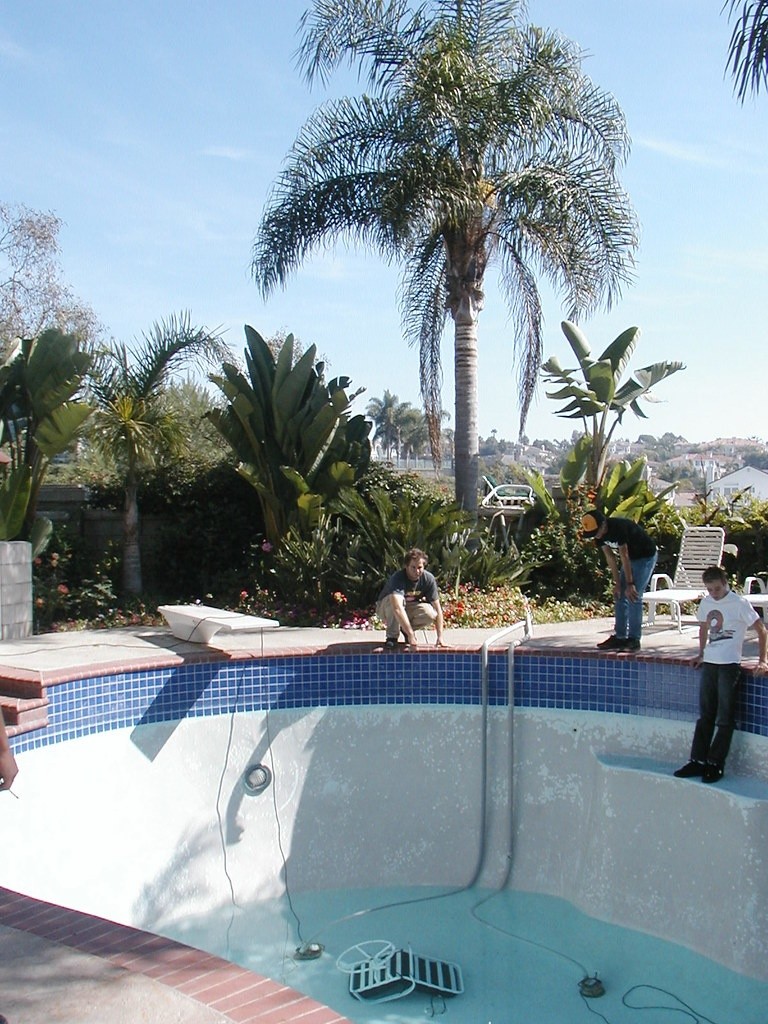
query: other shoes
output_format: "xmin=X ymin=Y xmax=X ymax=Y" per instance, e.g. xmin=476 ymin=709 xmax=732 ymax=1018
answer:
xmin=618 ymin=637 xmax=641 ymax=651
xmin=597 ymin=634 xmax=628 ymax=649
xmin=385 ymin=638 xmax=398 ymax=649
xmin=701 ymin=764 xmax=724 ymax=783
xmin=400 ymin=627 xmax=409 ymax=643
xmin=673 ymin=759 xmax=707 ymax=777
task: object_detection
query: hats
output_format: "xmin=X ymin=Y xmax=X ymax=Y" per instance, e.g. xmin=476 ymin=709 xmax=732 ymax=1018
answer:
xmin=580 ymin=509 xmax=605 ymax=538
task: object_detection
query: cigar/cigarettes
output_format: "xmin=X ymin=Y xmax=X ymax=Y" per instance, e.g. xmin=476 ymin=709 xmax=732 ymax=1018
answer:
xmin=1 ymin=781 xmax=19 ymax=799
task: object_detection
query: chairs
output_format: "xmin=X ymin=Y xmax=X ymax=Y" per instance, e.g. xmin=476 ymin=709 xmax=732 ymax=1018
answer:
xmin=742 ymin=577 xmax=768 ymax=624
xmin=642 ymin=527 xmax=726 ymax=636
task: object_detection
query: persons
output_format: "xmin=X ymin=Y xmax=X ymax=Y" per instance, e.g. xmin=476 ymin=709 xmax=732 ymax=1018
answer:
xmin=673 ymin=566 xmax=768 ymax=783
xmin=581 ymin=510 xmax=659 ymax=653
xmin=375 ymin=548 xmax=453 ymax=653
xmin=0 ymin=705 xmax=19 ymax=791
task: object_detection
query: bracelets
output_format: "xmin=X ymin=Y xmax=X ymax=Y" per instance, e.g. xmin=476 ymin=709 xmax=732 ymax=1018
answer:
xmin=759 ymin=659 xmax=767 ymax=663
xmin=627 ymin=582 xmax=635 ymax=586
